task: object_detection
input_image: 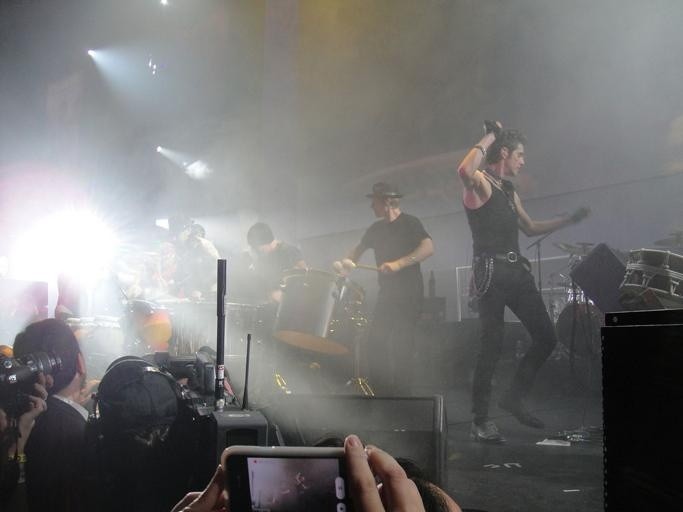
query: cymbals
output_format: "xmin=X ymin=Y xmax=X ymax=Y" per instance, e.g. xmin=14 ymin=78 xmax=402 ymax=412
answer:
xmin=654 ymin=238 xmax=682 ymax=247
xmin=553 ymin=241 xmax=595 ymax=257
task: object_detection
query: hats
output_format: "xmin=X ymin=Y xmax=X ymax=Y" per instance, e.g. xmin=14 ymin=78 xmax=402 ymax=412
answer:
xmin=363 ymin=180 xmax=407 ymax=201
xmin=246 ymin=222 xmax=275 ymax=246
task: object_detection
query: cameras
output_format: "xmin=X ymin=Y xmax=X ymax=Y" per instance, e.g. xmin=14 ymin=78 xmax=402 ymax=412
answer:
xmin=0 ymin=349 xmax=63 ymax=419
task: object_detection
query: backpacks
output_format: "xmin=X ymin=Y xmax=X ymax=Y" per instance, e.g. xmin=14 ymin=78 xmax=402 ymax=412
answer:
xmin=96 ymin=355 xmax=187 ymax=449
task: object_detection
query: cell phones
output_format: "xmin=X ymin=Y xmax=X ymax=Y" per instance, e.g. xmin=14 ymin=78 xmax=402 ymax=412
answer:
xmin=220 ymin=445 xmax=360 ymax=512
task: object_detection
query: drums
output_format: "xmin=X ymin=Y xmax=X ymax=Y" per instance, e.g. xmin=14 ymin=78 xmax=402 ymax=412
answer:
xmin=127 ymin=299 xmax=173 ymax=349
xmin=618 ymin=247 xmax=683 ymax=301
xmin=194 ymin=300 xmax=272 ymax=358
xmin=269 ymin=266 xmax=366 ymax=356
xmin=555 ymin=291 xmax=606 ymax=360
xmin=67 ymin=314 xmax=126 ymax=362
xmin=156 ymin=298 xmax=206 ymax=364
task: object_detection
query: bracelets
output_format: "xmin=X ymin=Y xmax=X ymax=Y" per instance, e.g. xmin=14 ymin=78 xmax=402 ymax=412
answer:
xmin=406 ymin=254 xmax=419 ymax=265
xmin=472 ymin=143 xmax=488 ymax=161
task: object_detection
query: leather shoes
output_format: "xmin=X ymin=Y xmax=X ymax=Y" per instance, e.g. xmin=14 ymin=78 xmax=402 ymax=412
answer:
xmin=469 ymin=422 xmax=508 ymax=445
xmin=499 ymin=394 xmax=547 ymax=429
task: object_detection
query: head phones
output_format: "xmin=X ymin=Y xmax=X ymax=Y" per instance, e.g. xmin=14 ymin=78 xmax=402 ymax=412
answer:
xmin=87 ymin=359 xmax=197 ymax=437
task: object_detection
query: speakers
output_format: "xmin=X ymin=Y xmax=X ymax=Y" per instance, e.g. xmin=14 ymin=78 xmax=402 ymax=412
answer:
xmin=277 ymin=393 xmax=448 ymax=491
xmin=600 ymin=325 xmax=683 ymax=511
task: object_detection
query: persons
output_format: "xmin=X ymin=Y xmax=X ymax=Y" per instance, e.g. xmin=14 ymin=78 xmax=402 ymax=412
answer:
xmin=455 ymin=119 xmax=595 ymax=444
xmin=393 ymin=454 xmax=464 ymax=512
xmin=242 ymin=222 xmax=312 ymax=343
xmin=327 ymin=180 xmax=436 ymax=395
xmin=171 ymin=434 xmax=426 ymax=512
